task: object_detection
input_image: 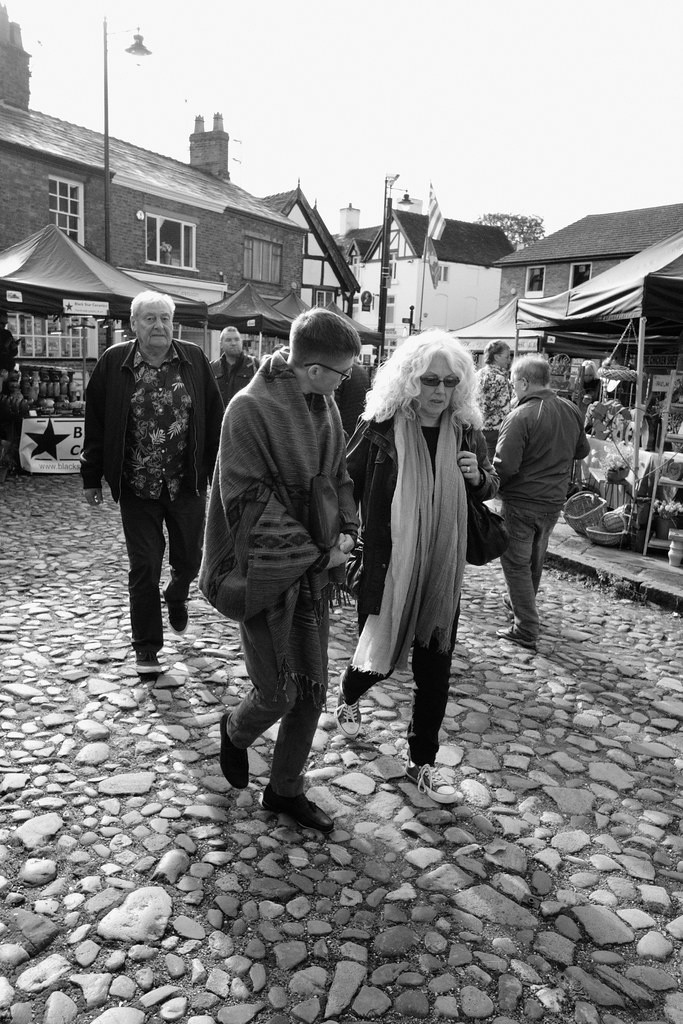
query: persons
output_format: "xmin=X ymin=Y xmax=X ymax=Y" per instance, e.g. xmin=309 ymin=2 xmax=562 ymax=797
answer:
xmin=493 ymin=353 xmax=591 ymax=647
xmin=572 ymin=360 xmax=603 ymax=414
xmin=334 ymin=362 xmax=371 ymax=438
xmin=198 ymin=308 xmax=362 ymax=834
xmin=340 ymin=328 xmax=501 ymax=803
xmin=80 ymin=289 xmax=225 ymax=674
xmin=0 ymin=309 xmax=18 ymax=371
xmin=209 ymin=327 xmax=259 ymax=408
xmin=475 ymin=341 xmax=516 ymax=465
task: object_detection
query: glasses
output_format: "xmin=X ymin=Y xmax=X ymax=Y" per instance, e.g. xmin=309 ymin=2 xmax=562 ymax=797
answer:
xmin=421 ymin=374 xmax=460 ymax=388
xmin=304 ymin=362 xmax=353 ymax=382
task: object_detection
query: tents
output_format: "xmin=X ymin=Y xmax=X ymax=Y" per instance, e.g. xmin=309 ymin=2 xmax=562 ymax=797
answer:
xmin=449 ymin=227 xmax=683 ymax=549
xmin=0 ymin=224 xmax=208 ymax=389
xmin=207 ymin=283 xmax=382 ymax=366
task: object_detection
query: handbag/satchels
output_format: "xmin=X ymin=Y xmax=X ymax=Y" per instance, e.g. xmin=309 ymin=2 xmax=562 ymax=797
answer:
xmin=312 ymin=473 xmax=342 ymax=550
xmin=337 ymin=535 xmax=364 ymax=597
xmin=467 ymin=488 xmax=509 ymax=566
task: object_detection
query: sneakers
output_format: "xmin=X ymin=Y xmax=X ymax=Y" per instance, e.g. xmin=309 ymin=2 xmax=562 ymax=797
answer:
xmin=262 ymin=783 xmax=334 ymax=833
xmin=135 ymin=651 xmax=161 ymax=674
xmin=502 ymin=594 xmax=513 ymax=612
xmin=334 ymin=671 xmax=362 ymax=738
xmin=496 ymin=625 xmax=536 ymax=646
xmin=405 ymin=757 xmax=459 ymax=804
xmin=219 ymin=713 xmax=250 ymax=789
xmin=162 ymin=589 xmax=189 ymax=636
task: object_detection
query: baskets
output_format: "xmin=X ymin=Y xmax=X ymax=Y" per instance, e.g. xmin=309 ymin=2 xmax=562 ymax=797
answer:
xmin=560 ymin=490 xmax=631 ymax=545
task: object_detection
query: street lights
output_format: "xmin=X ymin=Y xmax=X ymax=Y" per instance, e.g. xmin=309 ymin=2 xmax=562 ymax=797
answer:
xmin=98 ymin=12 xmax=155 ymax=263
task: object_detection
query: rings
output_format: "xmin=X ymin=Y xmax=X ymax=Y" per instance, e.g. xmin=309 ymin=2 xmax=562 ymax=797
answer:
xmin=467 ymin=466 xmax=470 ymax=472
